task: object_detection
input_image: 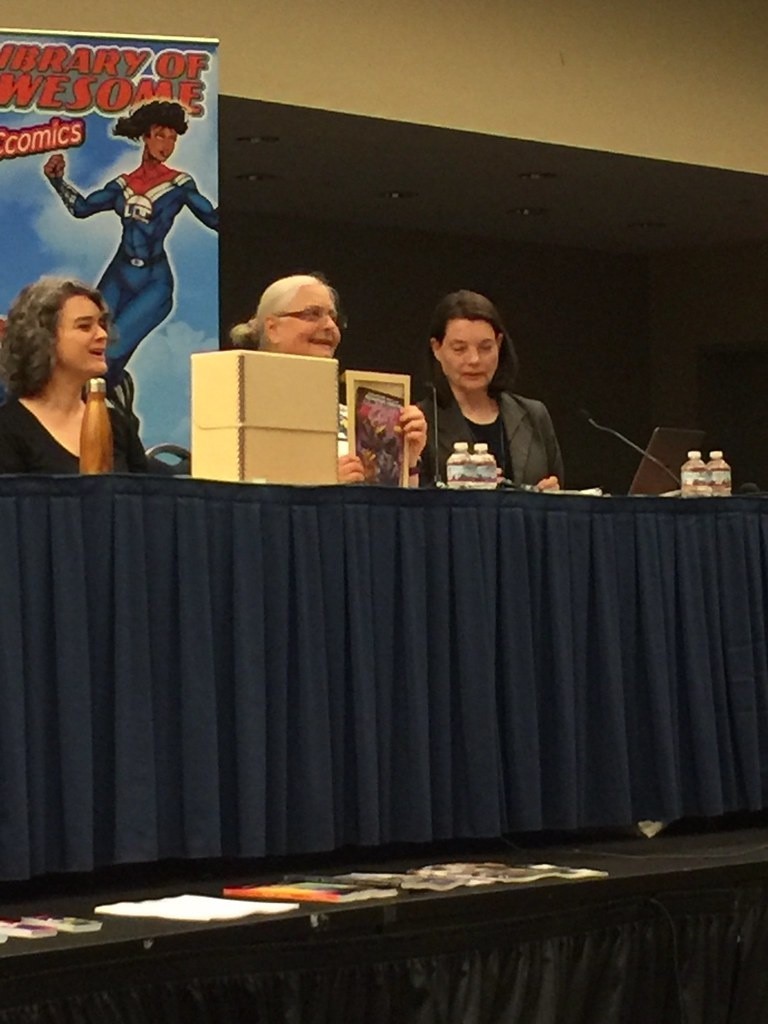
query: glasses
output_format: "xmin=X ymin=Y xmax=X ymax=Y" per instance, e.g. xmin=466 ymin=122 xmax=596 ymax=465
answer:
xmin=274 ymin=305 xmax=348 ymax=330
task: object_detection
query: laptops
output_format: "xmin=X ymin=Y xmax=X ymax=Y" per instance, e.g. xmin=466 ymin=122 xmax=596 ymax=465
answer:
xmin=627 ymin=427 xmax=706 ymax=496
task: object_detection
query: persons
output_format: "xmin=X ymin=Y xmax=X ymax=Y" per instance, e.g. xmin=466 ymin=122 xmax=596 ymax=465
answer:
xmin=413 ymin=288 xmax=565 ymax=491
xmin=0 ymin=274 xmax=149 ymax=475
xmin=230 ymin=273 xmax=427 ymax=490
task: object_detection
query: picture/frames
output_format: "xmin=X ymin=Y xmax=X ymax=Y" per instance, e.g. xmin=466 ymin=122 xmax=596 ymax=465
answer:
xmin=344 ymin=370 xmax=411 ymax=487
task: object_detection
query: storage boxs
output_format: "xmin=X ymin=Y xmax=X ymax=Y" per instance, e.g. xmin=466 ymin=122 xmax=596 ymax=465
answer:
xmin=189 ymin=349 xmax=341 ymax=485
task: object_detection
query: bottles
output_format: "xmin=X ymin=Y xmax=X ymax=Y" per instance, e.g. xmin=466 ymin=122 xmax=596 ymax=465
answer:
xmin=681 ymin=451 xmax=713 ymax=497
xmin=79 ymin=378 xmax=114 ymax=476
xmin=472 ymin=444 xmax=497 ymax=490
xmin=707 ymin=450 xmax=732 ymax=497
xmin=497 ymin=468 xmax=508 ymax=491
xmin=446 ymin=442 xmax=478 ymax=490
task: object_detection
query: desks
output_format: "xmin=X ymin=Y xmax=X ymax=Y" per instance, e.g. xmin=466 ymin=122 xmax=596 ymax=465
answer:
xmin=0 ymin=472 xmax=767 ymax=880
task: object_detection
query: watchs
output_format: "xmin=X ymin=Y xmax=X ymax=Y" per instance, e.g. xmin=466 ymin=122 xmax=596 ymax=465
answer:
xmin=408 ymin=456 xmax=422 ymax=476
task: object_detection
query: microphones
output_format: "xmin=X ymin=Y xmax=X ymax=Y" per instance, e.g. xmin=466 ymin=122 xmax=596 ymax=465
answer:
xmin=423 ymin=381 xmax=450 ymax=481
xmin=578 ymin=410 xmax=681 ymax=486
